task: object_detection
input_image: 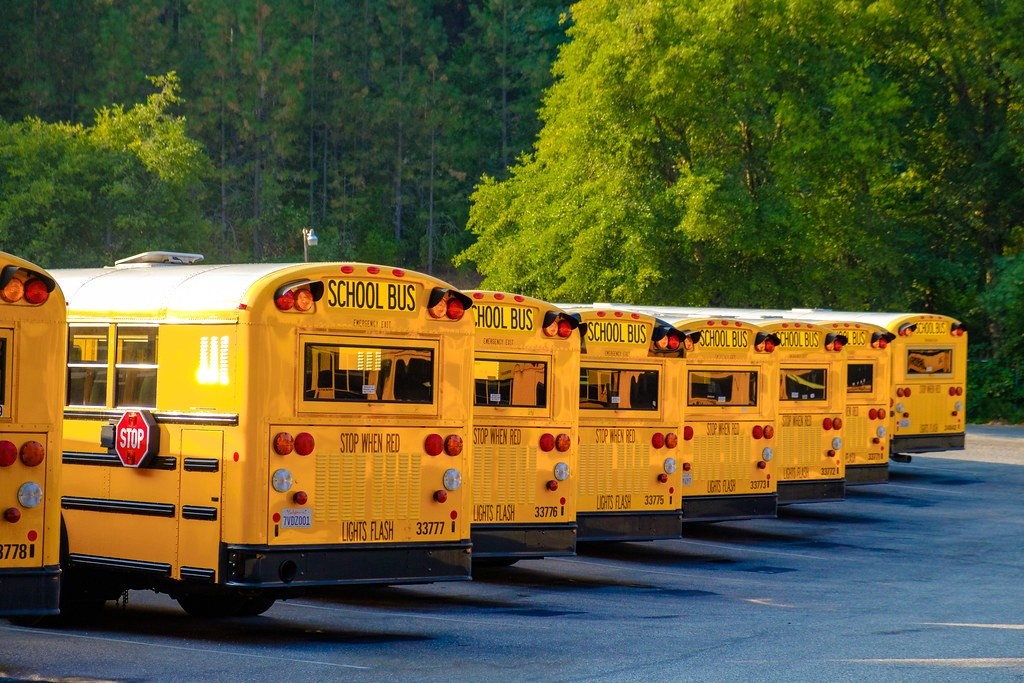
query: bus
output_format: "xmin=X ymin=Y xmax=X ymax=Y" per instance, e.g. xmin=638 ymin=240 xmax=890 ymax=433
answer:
xmin=305 ymin=288 xmax=582 ymax=575
xmin=44 ymin=250 xmax=476 ymax=623
xmin=1 ymin=252 xmax=68 ymax=618
xmin=554 ymin=301 xmax=969 ymax=540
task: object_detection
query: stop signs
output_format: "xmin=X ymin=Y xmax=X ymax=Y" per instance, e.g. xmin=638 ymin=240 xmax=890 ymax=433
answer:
xmin=114 ymin=408 xmax=161 ymax=469
